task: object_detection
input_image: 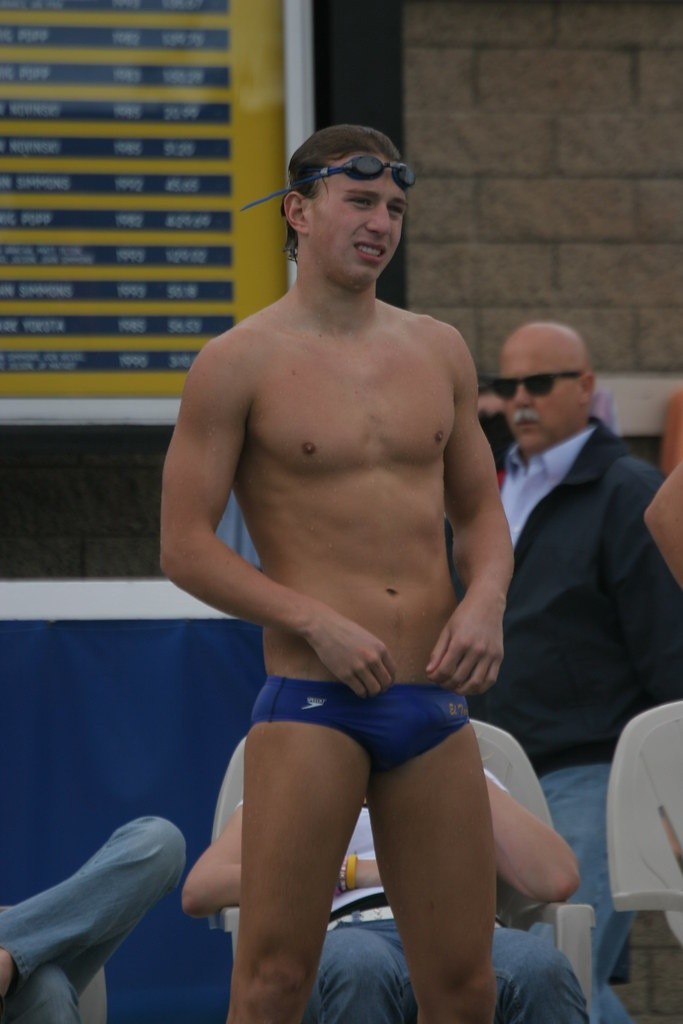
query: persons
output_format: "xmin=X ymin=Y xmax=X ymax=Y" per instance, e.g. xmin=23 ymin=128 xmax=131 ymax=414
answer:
xmin=0 ymin=817 xmax=187 ymax=1024
xmin=179 ymin=763 xmax=590 ymax=1024
xmin=445 ymin=317 xmax=683 ymax=1024
xmin=158 ymin=126 xmax=514 ymax=1024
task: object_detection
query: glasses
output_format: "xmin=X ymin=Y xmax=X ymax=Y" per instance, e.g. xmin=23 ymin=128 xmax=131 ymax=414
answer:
xmin=490 ymin=371 xmax=581 ymax=399
xmin=344 ymin=155 xmax=416 ymax=190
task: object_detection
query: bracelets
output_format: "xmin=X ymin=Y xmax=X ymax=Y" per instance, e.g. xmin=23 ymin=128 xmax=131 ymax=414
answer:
xmin=334 ymin=855 xmax=356 ymax=895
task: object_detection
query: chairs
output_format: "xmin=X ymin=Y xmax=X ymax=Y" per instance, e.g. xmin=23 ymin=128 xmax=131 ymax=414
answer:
xmin=208 ymin=715 xmax=595 ymax=1024
